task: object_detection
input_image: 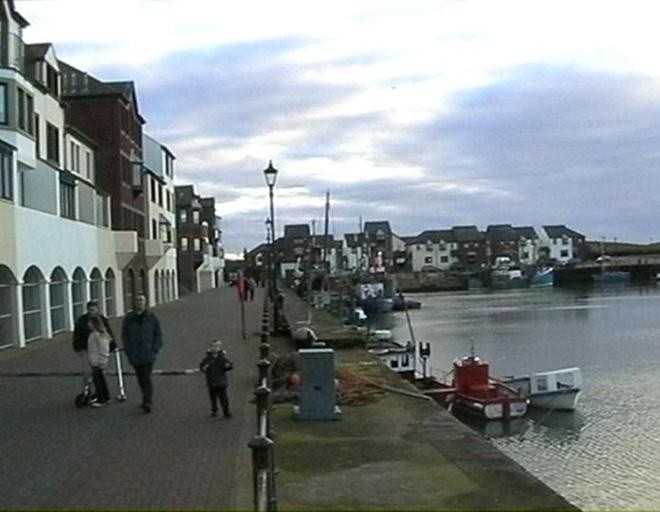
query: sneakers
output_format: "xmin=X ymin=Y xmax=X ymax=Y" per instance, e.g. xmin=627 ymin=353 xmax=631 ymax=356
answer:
xmin=141 ymin=400 xmax=153 ymax=413
xmin=89 ymin=397 xmax=112 ymax=407
xmin=212 ymin=410 xmax=232 ymax=417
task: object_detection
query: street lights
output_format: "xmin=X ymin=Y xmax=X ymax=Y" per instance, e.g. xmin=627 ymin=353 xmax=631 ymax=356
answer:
xmin=265 ymin=217 xmax=272 ymax=290
xmin=263 ymin=158 xmax=278 ymax=295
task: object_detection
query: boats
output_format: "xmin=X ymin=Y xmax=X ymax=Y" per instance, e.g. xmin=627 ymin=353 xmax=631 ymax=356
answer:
xmin=367 ymin=340 xmax=455 ymax=408
xmin=444 ymin=337 xmax=527 ymax=419
xmin=342 ymin=319 xmax=393 ymax=339
xmin=499 ymin=366 xmax=585 ymax=411
xmin=355 ymin=295 xmax=396 ymax=314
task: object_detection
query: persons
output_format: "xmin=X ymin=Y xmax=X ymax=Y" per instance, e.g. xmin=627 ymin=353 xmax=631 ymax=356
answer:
xmin=73 ymin=301 xmax=119 ymax=401
xmin=120 ymin=290 xmax=164 ymax=410
xmin=199 ymin=339 xmax=235 ymax=417
xmin=243 ymin=273 xmax=249 ymax=301
xmin=85 ymin=314 xmax=114 ymax=408
xmin=248 ymin=276 xmax=258 ymax=301
